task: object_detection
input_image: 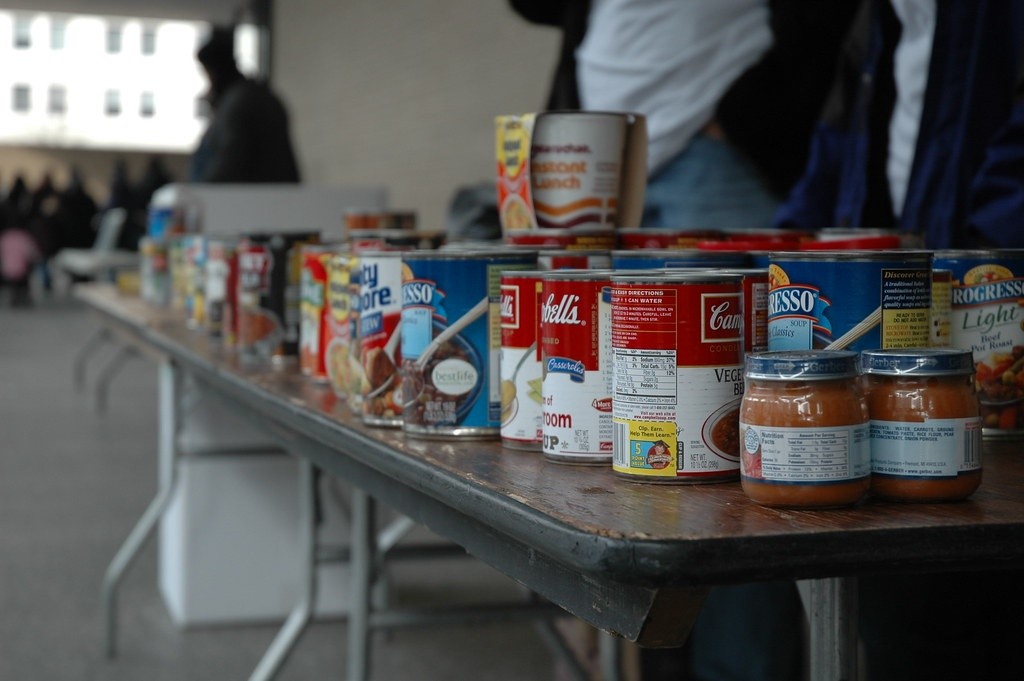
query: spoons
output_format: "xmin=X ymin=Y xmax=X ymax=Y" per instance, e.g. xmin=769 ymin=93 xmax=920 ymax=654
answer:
xmin=365 ymin=315 xmax=407 ymax=398
xmin=404 ymin=298 xmax=489 ymax=408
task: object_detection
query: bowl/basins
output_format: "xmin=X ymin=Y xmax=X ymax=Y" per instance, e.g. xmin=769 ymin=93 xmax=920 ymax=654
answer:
xmin=403 ymin=316 xmax=480 ymax=425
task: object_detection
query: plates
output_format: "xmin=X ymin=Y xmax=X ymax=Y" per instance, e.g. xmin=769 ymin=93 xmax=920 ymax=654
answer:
xmin=972 ymin=353 xmax=1024 ymax=407
xmin=702 ymin=398 xmax=744 ymax=463
xmin=500 ymin=377 xmax=519 ymax=428
xmin=324 ymin=336 xmax=355 ymax=400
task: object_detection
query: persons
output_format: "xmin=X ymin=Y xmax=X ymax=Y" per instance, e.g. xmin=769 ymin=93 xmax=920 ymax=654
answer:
xmin=0 ymin=154 xmax=179 ymax=307
xmin=190 ymin=35 xmax=302 ymax=185
xmin=505 ymin=0 xmax=1024 ymax=681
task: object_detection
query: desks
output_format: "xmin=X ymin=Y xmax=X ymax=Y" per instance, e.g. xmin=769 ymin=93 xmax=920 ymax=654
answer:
xmin=74 ymin=283 xmax=1023 ymax=681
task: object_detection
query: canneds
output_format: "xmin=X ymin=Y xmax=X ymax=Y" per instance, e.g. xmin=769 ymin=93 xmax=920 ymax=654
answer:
xmin=174 ymin=109 xmax=1023 ymax=507
